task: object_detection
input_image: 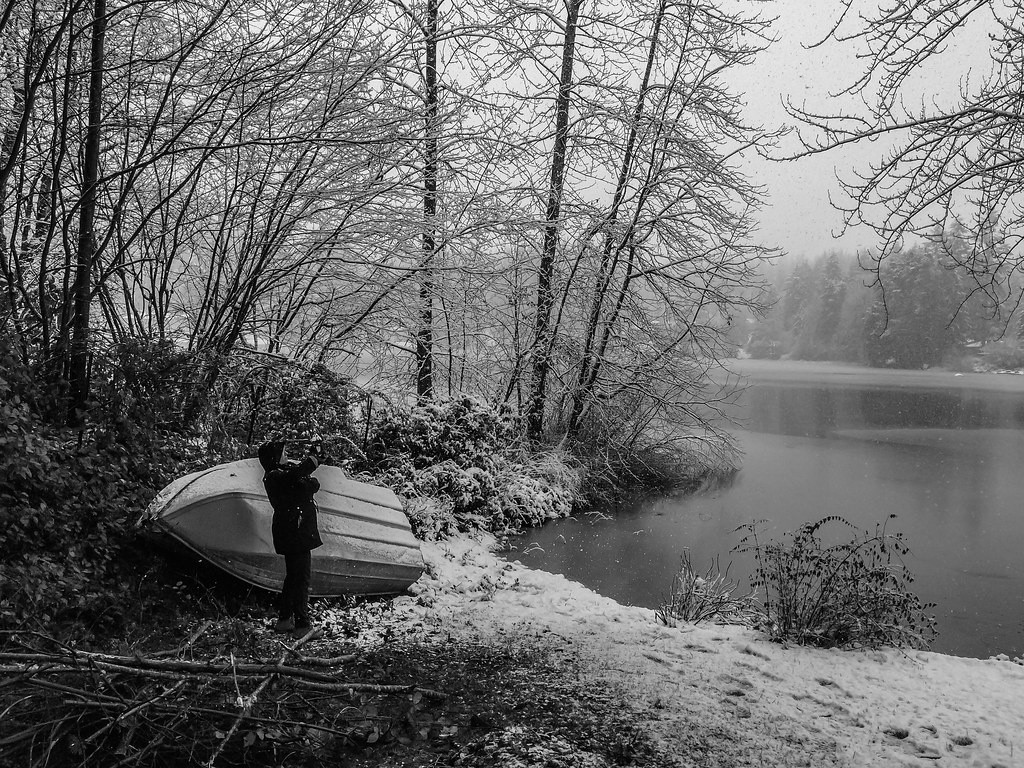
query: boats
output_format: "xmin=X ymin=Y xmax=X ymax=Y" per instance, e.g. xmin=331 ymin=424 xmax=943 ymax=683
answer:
xmin=133 ymin=458 xmax=426 ymax=604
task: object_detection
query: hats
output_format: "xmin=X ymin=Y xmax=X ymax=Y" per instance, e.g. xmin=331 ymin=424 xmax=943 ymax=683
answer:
xmin=258 ymin=441 xmax=286 ymax=465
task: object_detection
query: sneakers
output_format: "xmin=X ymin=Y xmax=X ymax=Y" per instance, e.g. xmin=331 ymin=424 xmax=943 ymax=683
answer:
xmin=274 ymin=619 xmax=296 ymax=633
xmin=293 ymin=625 xmax=325 ymax=640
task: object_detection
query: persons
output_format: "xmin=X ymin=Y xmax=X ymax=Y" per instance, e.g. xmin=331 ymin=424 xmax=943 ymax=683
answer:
xmin=258 ymin=441 xmax=326 ymax=639
